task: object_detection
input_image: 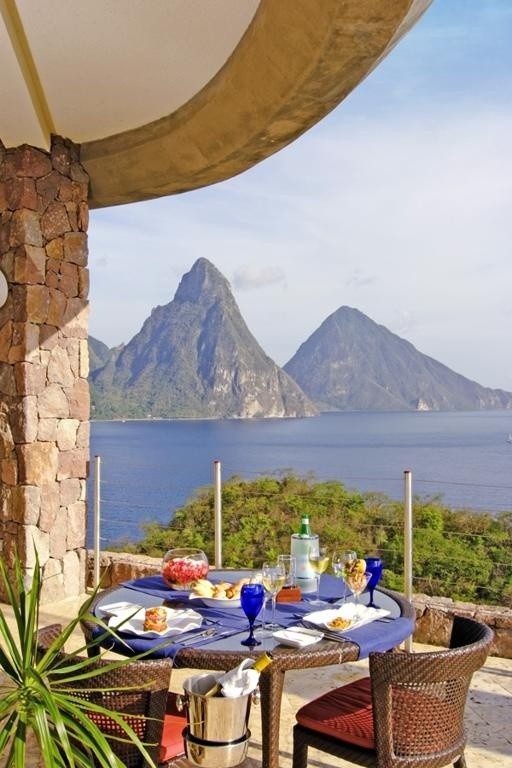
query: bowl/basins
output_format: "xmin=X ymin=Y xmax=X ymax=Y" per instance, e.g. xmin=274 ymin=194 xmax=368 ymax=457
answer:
xmin=182 ymin=674 xmax=251 ymax=740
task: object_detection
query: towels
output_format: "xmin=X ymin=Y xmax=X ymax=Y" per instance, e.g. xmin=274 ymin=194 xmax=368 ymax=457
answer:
xmin=215 ymin=657 xmax=261 ymax=699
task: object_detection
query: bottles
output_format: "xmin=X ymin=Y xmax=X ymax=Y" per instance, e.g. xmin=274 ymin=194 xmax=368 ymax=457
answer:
xmin=296 ymin=514 xmax=312 ymax=537
xmin=205 ymin=651 xmax=274 ymax=697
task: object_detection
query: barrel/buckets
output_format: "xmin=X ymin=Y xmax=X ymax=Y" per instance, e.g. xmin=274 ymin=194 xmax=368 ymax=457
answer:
xmin=176 ymin=674 xmax=260 ymax=741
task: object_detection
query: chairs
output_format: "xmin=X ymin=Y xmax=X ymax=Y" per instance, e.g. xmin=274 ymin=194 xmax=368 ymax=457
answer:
xmin=293 ymin=614 xmax=494 ymax=768
xmin=31 ymin=623 xmax=188 ymax=768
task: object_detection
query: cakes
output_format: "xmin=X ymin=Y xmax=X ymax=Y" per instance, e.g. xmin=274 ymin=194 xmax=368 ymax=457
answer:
xmin=144 ymin=608 xmax=168 ymax=631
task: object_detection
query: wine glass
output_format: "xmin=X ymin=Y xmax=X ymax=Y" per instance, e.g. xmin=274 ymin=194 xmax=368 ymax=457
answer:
xmin=240 ymin=582 xmax=262 ymax=646
xmin=309 ymin=545 xmax=330 ymax=605
xmin=330 ymin=549 xmax=352 ymax=604
xmin=362 ymin=556 xmax=381 ymax=607
xmin=276 ymin=555 xmax=294 ymax=587
xmin=343 ymin=572 xmax=373 ymax=603
xmin=262 ymin=561 xmax=281 ymax=628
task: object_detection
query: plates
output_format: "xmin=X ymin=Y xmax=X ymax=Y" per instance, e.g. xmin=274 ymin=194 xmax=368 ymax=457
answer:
xmin=271 ymin=626 xmax=323 ymax=648
xmin=108 ymin=607 xmax=203 ymax=638
xmin=189 ymin=592 xmax=242 ymax=609
xmin=303 ymin=604 xmax=391 ymax=631
